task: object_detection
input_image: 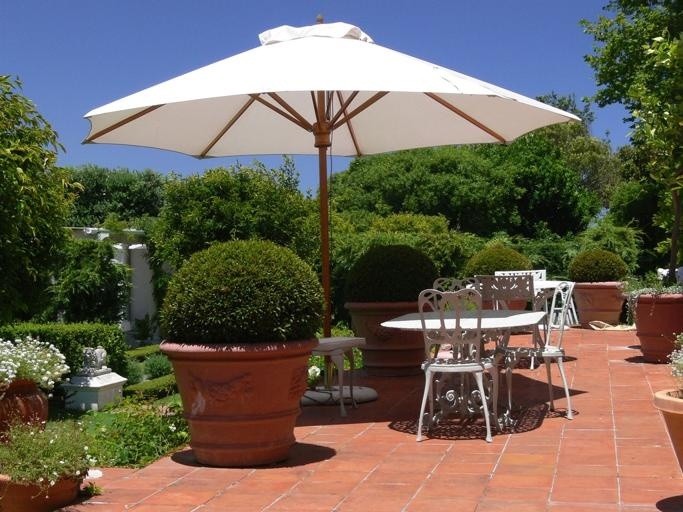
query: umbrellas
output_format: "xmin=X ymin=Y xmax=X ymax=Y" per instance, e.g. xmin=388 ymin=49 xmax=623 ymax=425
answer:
xmin=81 ymin=13 xmax=586 ymax=387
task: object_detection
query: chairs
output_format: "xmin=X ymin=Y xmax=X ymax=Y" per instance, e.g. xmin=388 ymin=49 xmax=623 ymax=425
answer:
xmin=416 ymin=270 xmax=578 ymax=441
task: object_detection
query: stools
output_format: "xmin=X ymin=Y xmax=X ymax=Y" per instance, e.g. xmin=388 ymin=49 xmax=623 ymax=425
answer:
xmin=305 ymin=336 xmax=363 ymax=416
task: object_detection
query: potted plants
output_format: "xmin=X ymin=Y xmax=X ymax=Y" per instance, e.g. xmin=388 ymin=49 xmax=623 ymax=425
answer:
xmin=155 ymin=239 xmax=330 ymax=471
xmin=343 ymin=242 xmax=442 ymax=378
xmin=623 ymin=269 xmax=683 ymax=471
xmin=0 ymin=436 xmax=94 ymax=511
xmin=464 ymin=250 xmax=630 ymax=329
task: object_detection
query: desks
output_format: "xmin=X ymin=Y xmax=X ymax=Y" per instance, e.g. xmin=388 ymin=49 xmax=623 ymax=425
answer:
xmin=378 ymin=308 xmax=548 ymax=414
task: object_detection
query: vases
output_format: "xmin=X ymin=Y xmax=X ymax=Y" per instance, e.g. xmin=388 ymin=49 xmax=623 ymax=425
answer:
xmin=0 ymin=381 xmax=49 ymax=443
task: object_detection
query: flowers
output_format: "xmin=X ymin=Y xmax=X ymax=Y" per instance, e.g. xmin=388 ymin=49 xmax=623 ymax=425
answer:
xmin=0 ymin=337 xmax=71 ymax=398
xmin=307 ymin=365 xmax=320 ymax=389
